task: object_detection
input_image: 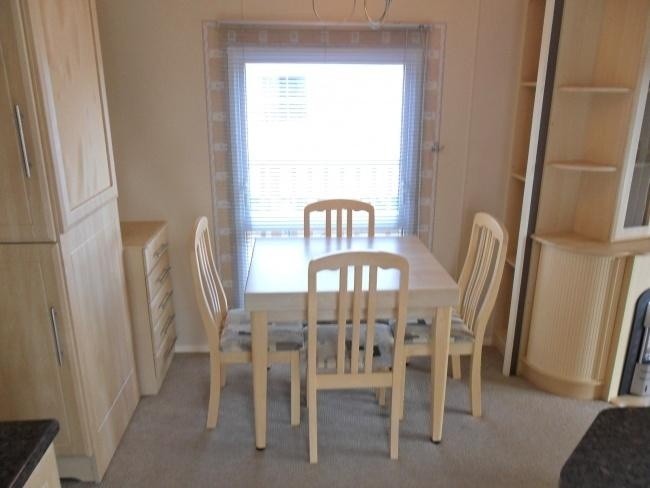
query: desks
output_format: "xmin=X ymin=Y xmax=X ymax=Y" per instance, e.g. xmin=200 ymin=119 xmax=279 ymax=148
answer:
xmin=1 ymin=419 xmax=61 ymax=487
xmin=245 ymin=234 xmax=460 ymax=464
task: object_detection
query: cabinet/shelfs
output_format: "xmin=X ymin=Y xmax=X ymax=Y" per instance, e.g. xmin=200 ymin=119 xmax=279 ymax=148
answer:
xmin=501 ymin=2 xmax=649 ymax=405
xmin=1 ymin=1 xmax=132 ymax=467
xmin=121 ymin=217 xmax=176 ymax=406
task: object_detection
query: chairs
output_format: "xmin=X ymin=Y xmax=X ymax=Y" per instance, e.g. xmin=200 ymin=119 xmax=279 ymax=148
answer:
xmin=302 ymin=195 xmax=387 ymax=407
xmin=302 ymin=246 xmax=414 ymax=467
xmin=192 ymin=216 xmax=300 ymax=434
xmin=409 ymin=212 xmax=512 ymax=429
